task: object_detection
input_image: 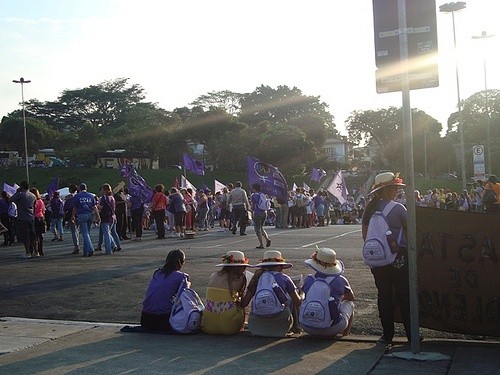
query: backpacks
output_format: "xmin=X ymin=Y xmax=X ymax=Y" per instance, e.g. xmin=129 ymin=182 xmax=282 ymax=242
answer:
xmin=249 ymin=272 xmax=285 ymax=317
xmin=255 ymin=193 xmax=271 ymax=210
xmin=169 ymin=273 xmax=206 ymax=334
xmin=485 ymin=189 xmax=498 ymax=202
xmin=361 ymin=201 xmax=403 ymax=267
xmin=295 ymin=195 xmax=304 ymax=207
xmin=478 ymin=186 xmax=485 ymax=198
xmin=299 ymin=275 xmax=344 ymax=328
xmin=57 ymin=205 xmax=65 ymax=218
xmin=8 ymin=202 xmax=18 ymax=218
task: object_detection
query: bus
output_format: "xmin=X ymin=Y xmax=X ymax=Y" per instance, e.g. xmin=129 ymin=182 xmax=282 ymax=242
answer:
xmin=94 ymin=150 xmax=160 ymax=170
xmin=0 ymin=151 xmax=19 ymax=167
xmin=35 ymin=148 xmax=56 ymax=161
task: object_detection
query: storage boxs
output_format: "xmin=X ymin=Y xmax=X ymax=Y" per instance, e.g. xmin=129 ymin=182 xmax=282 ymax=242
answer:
xmin=185 ymin=231 xmax=195 ymax=238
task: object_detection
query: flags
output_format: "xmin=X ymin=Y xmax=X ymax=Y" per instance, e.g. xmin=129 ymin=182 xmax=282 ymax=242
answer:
xmin=312 ymin=168 xmax=322 ymax=182
xmin=245 ymin=154 xmax=288 ymax=203
xmin=183 ymin=153 xmax=205 ymax=176
xmin=47 ymin=178 xmax=58 ymax=195
xmin=4 ymin=184 xmax=16 ymax=195
xmin=128 ymin=170 xmax=154 ymax=204
xmin=326 ymin=170 xmax=349 ymax=204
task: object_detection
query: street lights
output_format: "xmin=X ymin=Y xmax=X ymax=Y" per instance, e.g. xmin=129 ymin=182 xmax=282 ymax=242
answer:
xmin=439 ymin=1 xmax=466 ymax=197
xmin=472 ymin=31 xmax=495 ymax=176
xmin=12 ymin=78 xmax=31 ymax=190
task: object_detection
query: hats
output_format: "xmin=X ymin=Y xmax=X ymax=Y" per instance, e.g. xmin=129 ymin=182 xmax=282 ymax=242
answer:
xmin=208 ymin=189 xmax=211 ymax=192
xmin=253 ymin=184 xmax=261 ymax=191
xmin=215 ymin=251 xmax=253 ymax=268
xmin=79 ymin=183 xmax=86 ymax=190
xmin=254 ymin=250 xmax=293 ymax=269
xmin=199 ymin=189 xmax=204 ymax=192
xmin=304 ymin=245 xmax=345 ymax=276
xmin=366 ymin=172 xmax=406 ymax=196
xmin=236 ymin=181 xmax=242 ymax=187
xmin=398 ymin=189 xmax=405 ymax=195
xmin=224 ymin=187 xmax=227 ymax=191
xmin=318 ymin=188 xmax=324 ymax=192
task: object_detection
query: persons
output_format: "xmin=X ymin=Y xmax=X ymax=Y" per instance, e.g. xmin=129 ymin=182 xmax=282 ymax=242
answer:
xmin=298 ymin=248 xmax=355 ymax=336
xmin=200 ymin=251 xmax=253 ymax=334
xmin=140 ymin=249 xmax=191 ymax=327
xmin=44 ymin=183 xmax=121 ymax=257
xmin=113 ymin=181 xmax=250 ymax=241
xmin=250 ymin=184 xmax=271 ymax=249
xmin=0 ymin=181 xmax=46 ymax=258
xmin=265 ymin=188 xmax=366 ymax=227
xmin=362 ymin=172 xmax=424 ymax=343
xmin=414 ymin=175 xmax=500 ymax=214
xmin=243 ymin=250 xmax=301 ymax=336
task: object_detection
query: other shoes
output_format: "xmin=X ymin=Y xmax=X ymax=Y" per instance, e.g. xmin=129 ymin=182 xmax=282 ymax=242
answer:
xmin=3 ymin=233 xmax=166 ymax=258
xmin=408 ymin=337 xmax=423 ymax=342
xmin=168 ymin=227 xmax=247 ymax=237
xmin=256 ymin=246 xmax=264 ymax=249
xmin=379 ymin=338 xmax=392 ymax=344
xmin=267 ymin=240 xmax=271 ymax=247
